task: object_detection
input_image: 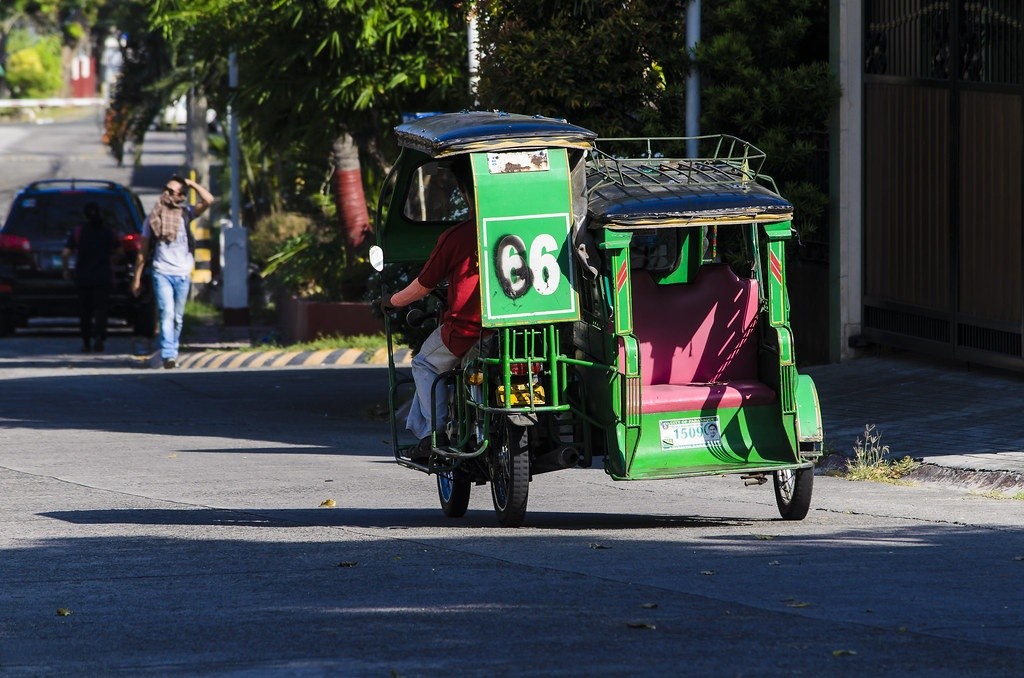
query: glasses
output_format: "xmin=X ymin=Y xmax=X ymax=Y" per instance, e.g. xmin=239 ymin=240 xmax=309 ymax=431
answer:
xmin=163 ymin=185 xmax=174 ymax=195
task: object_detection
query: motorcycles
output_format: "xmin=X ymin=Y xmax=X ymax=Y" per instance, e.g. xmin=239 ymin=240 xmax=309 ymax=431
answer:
xmin=369 ymin=103 xmax=827 ymax=531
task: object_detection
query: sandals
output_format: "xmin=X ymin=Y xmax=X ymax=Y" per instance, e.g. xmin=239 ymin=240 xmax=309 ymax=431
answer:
xmin=409 ymin=433 xmax=450 ymax=459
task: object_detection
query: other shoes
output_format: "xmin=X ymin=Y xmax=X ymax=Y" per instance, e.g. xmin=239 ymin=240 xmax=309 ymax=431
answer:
xmin=165 ymin=358 xmax=175 ymax=368
xmin=94 ymin=341 xmax=105 ymax=352
xmin=82 ymin=345 xmax=92 ymax=353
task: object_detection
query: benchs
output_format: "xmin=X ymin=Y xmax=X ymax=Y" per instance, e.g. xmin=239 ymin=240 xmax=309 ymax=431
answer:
xmin=610 ymin=262 xmax=778 ymax=415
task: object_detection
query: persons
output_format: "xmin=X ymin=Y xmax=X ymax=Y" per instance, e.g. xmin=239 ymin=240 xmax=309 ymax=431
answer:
xmin=129 ymin=176 xmax=215 ymax=366
xmin=379 ymin=169 xmax=498 ymax=464
xmin=62 ymin=202 xmax=122 ymax=352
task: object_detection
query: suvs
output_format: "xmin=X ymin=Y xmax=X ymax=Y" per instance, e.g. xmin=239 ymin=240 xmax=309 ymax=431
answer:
xmin=1 ymin=179 xmax=154 ymax=341
